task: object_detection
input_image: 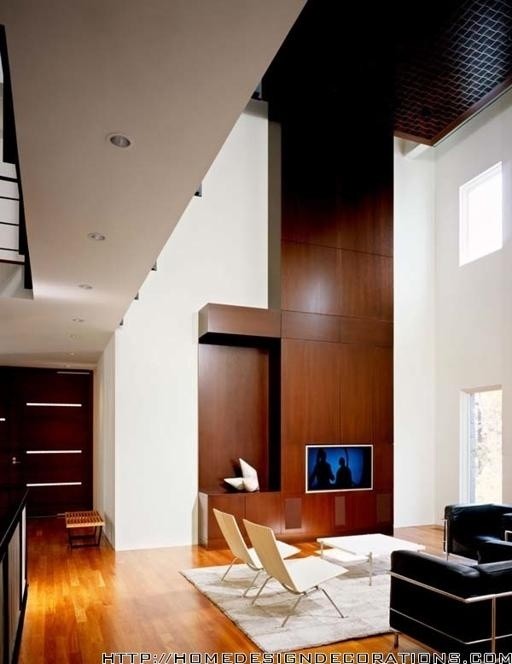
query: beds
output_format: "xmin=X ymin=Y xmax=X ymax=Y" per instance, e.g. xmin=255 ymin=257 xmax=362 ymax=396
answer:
xmin=315 ymin=533 xmax=425 ymax=586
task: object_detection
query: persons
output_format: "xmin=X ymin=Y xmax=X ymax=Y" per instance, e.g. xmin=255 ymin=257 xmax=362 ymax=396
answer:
xmin=334 ymin=457 xmax=352 ymax=489
xmin=309 ymin=449 xmax=335 ymax=489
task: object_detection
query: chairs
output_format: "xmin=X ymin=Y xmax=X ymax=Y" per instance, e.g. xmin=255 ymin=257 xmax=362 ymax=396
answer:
xmin=210 ymin=507 xmax=349 ymax=626
xmin=389 ymin=505 xmax=512 ymax=664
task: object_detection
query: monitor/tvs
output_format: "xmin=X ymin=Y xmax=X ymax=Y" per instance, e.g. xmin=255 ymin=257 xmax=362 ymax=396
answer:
xmin=305 ymin=444 xmax=373 ymax=493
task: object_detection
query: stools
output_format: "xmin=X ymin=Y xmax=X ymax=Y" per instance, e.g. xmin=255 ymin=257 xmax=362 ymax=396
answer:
xmin=64 ymin=510 xmax=105 ymax=548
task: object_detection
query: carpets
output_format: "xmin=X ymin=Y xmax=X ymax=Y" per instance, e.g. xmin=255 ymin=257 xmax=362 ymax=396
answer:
xmin=177 ymin=543 xmax=462 ymax=654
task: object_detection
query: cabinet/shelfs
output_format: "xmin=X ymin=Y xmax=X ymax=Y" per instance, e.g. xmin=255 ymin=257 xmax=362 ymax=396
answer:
xmin=0 ymin=497 xmax=27 ymax=664
